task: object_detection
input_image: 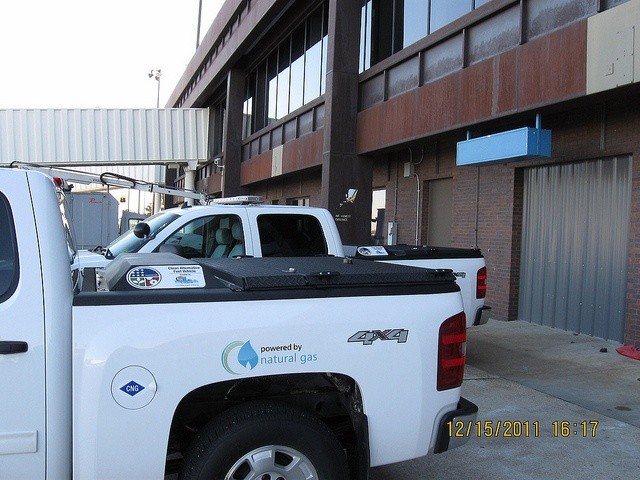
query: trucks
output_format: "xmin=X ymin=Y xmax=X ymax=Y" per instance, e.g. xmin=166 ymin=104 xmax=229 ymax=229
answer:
xmin=62 ymin=191 xmax=149 ymax=252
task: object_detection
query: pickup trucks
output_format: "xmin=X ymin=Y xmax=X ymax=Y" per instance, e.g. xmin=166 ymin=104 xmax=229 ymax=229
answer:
xmin=0 ymin=167 xmax=479 ymax=480
xmin=1 ymin=156 xmax=491 ymax=332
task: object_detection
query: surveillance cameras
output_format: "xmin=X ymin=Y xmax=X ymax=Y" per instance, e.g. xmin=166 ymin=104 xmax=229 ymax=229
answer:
xmin=214 ymin=158 xmax=221 ymax=165
